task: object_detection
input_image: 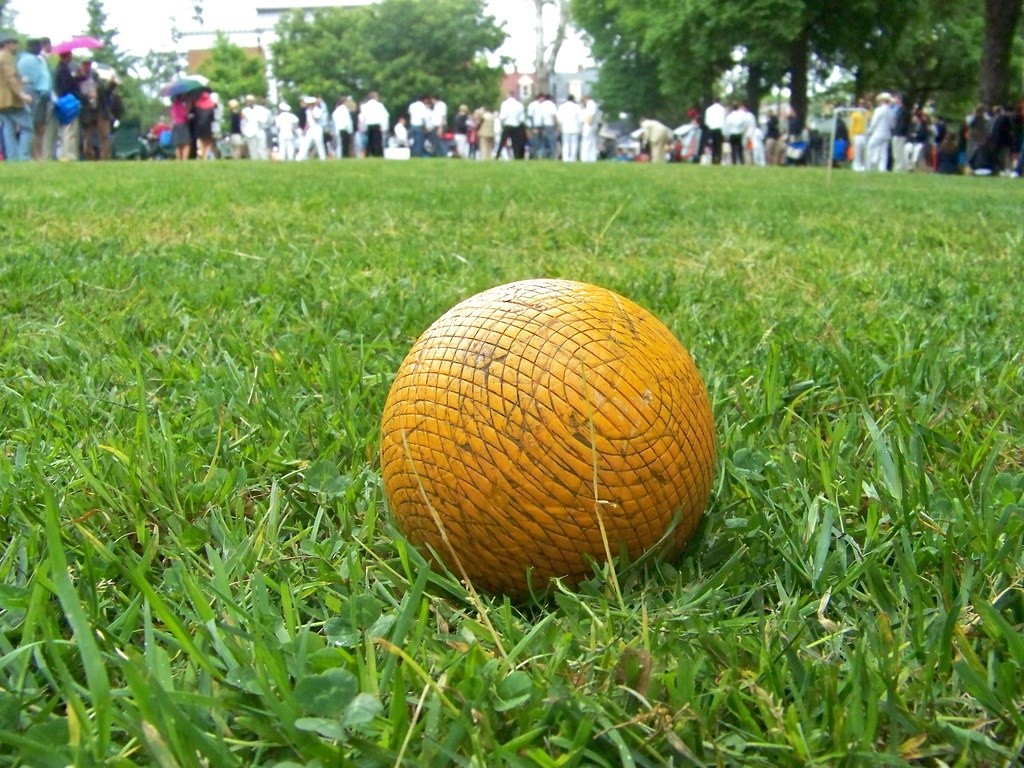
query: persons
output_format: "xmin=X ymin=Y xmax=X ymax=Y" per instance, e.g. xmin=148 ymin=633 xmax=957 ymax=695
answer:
xmin=394 ymin=91 xmax=810 ymax=167
xmin=0 ymin=36 xmax=123 ymax=160
xmin=146 ymin=87 xmax=389 ymax=160
xmin=833 ymin=91 xmax=1024 ymax=179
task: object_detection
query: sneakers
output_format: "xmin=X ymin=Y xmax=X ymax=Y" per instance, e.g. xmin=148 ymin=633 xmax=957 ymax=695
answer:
xmin=840 ymin=163 xmax=936 ymax=172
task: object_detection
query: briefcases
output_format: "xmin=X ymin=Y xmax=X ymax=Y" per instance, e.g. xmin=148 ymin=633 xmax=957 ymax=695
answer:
xmin=53 ymin=94 xmax=82 ymax=124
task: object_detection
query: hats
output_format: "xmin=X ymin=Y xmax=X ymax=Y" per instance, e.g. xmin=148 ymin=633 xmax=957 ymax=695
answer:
xmin=227 ymin=98 xmax=241 ymax=110
xmin=458 ymin=104 xmax=469 ymax=111
xmin=304 ymin=96 xmax=316 ymax=104
xmin=246 ymin=95 xmax=255 ymax=101
xmin=876 ymin=92 xmax=896 ymax=103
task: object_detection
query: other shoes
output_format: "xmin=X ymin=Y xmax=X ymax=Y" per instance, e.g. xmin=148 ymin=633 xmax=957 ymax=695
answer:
xmin=968 ymin=167 xmax=1020 ymax=179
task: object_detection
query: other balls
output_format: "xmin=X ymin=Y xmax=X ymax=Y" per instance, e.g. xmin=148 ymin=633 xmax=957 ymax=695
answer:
xmin=377 ymin=273 xmax=720 ymax=624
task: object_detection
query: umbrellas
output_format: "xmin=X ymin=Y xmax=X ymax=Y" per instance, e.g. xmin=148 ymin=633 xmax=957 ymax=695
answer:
xmin=159 ymin=74 xmax=209 ymax=97
xmin=52 ymin=36 xmax=103 ymax=56
